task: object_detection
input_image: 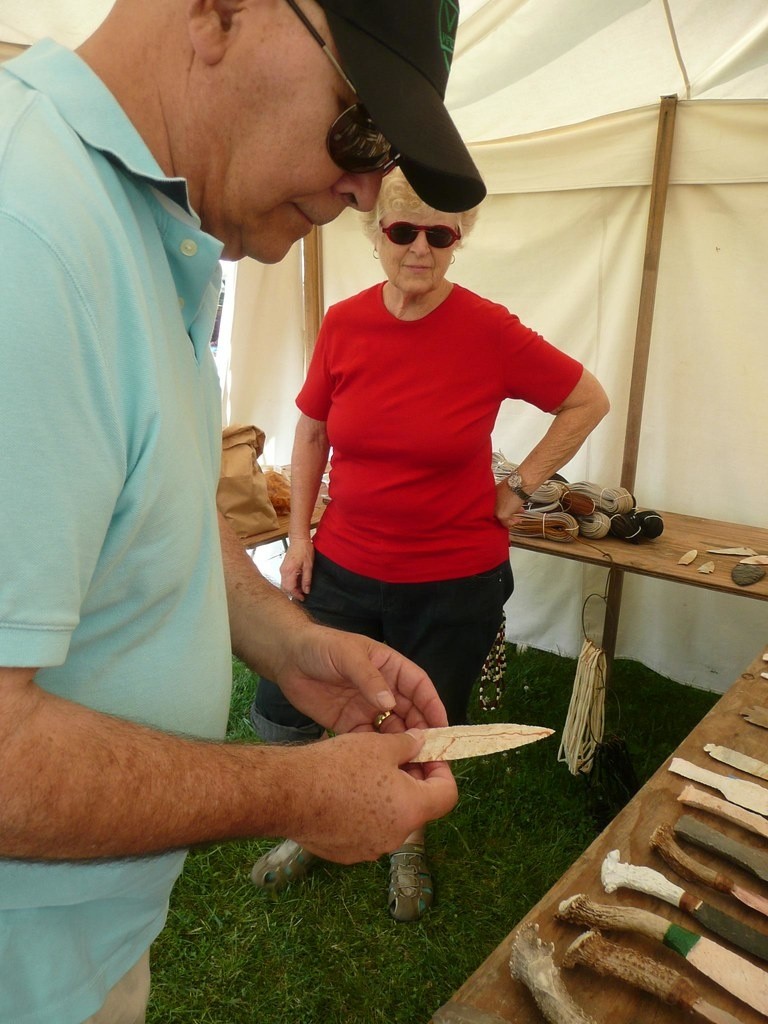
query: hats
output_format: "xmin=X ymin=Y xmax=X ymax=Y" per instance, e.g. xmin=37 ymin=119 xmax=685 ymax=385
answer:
xmin=319 ymin=0 xmax=487 ymax=212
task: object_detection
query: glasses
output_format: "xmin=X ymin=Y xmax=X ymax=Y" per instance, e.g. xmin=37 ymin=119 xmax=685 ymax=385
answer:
xmin=287 ymin=0 xmax=402 ymax=177
xmin=378 ymin=221 xmax=462 ymax=249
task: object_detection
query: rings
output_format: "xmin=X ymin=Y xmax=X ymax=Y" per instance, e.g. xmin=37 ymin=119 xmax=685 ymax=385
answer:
xmin=373 ymin=709 xmax=395 ymax=729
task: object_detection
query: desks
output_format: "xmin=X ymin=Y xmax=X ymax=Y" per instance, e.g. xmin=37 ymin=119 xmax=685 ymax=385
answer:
xmin=495 ymin=503 xmax=768 ymax=605
xmin=430 ymin=638 xmax=768 ymax=1024
xmin=238 ymin=453 xmax=335 ymax=558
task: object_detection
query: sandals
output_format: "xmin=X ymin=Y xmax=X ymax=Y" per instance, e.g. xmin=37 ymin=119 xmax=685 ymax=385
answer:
xmin=250 ymin=838 xmax=316 ymax=889
xmin=387 ymin=843 xmax=433 ymax=921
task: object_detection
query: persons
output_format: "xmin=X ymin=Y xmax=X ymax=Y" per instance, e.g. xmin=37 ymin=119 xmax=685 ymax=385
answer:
xmin=0 ymin=0 xmax=485 ymax=1024
xmin=251 ymin=163 xmax=610 ymax=920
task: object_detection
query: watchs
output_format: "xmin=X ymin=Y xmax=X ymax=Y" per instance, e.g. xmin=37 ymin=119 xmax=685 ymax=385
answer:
xmin=506 ymin=471 xmax=531 ymax=501
